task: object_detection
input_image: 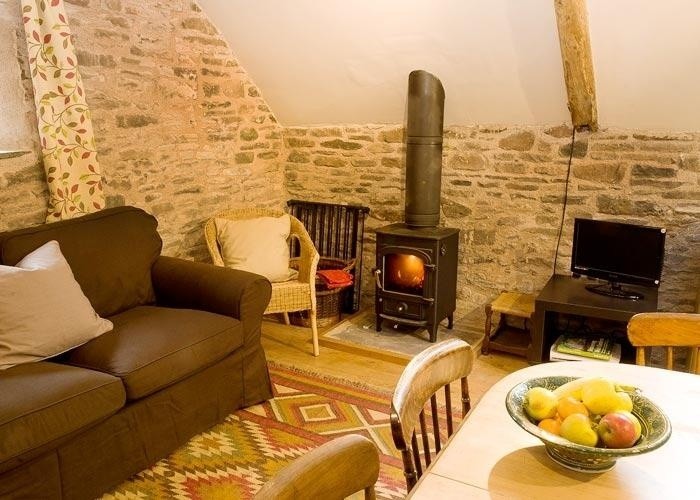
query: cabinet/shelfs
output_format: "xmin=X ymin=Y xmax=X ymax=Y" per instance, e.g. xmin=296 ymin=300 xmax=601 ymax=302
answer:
xmin=530 ymin=275 xmax=657 ymax=366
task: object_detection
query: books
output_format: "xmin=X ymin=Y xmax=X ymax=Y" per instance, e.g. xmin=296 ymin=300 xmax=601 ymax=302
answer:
xmin=550 ymin=333 xmax=622 ymax=367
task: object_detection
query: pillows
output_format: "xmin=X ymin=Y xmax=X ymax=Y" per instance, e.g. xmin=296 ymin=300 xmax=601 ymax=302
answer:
xmin=1 ymin=239 xmax=116 ymax=371
xmin=213 ymin=215 xmax=297 ymax=285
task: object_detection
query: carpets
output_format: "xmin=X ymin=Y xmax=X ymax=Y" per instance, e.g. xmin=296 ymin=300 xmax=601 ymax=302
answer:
xmin=92 ymin=359 xmax=466 ymax=500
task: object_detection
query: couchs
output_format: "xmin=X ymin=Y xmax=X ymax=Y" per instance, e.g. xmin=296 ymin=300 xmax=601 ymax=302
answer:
xmin=0 ymin=204 xmax=280 ymax=500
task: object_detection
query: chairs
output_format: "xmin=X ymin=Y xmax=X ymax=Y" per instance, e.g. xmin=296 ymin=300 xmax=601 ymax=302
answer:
xmin=628 ymin=312 xmax=699 ymax=377
xmin=389 ymin=338 xmax=477 ymax=493
xmin=204 ymin=206 xmax=320 ymax=357
xmin=253 ymin=434 xmax=380 ymax=500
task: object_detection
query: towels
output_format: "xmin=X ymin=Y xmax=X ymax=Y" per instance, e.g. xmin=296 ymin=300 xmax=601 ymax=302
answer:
xmin=318 ymin=270 xmax=353 ymax=289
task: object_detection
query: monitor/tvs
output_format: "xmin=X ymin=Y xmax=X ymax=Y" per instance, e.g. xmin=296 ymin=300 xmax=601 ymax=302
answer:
xmin=571 ymin=218 xmax=666 ymax=299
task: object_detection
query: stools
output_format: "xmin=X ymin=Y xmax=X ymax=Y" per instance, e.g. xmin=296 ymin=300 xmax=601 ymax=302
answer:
xmin=480 ymin=290 xmax=540 ymax=359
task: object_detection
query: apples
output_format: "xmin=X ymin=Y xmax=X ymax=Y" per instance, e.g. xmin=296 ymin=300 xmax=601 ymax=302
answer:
xmin=523 ymin=376 xmax=641 ymax=448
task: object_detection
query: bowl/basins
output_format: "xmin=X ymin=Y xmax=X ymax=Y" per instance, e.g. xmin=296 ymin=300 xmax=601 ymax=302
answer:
xmin=505 ymin=375 xmax=672 ymax=475
